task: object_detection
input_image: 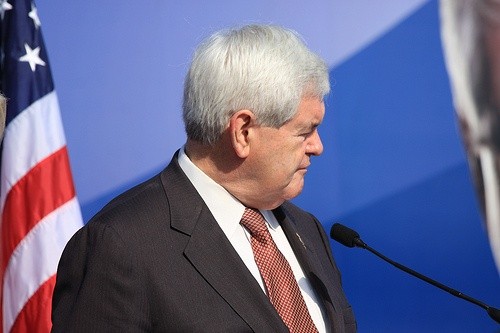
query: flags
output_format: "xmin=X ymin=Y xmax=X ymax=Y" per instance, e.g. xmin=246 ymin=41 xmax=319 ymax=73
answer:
xmin=0 ymin=0 xmax=85 ymax=333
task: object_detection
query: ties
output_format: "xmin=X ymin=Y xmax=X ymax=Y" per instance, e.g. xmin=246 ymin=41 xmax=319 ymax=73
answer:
xmin=239 ymin=207 xmax=320 ymax=333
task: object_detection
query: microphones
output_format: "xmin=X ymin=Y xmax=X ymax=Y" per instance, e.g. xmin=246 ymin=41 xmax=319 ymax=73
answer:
xmin=330 ymin=223 xmax=500 ymax=324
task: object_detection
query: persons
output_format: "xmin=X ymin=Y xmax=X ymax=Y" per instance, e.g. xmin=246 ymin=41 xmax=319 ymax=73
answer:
xmin=51 ymin=23 xmax=358 ymax=333
